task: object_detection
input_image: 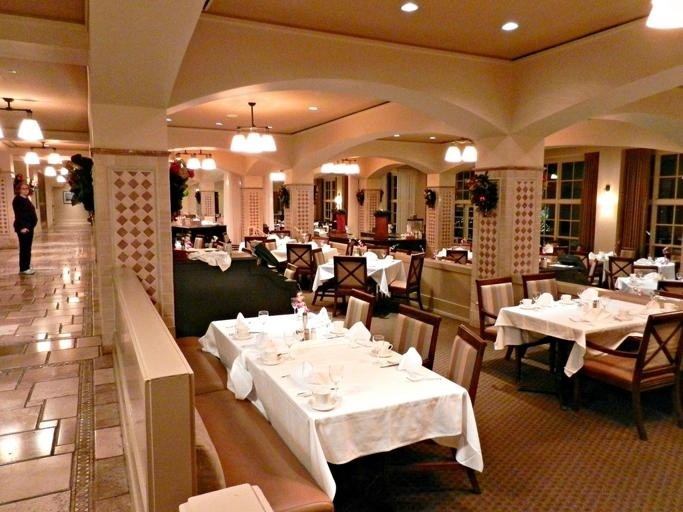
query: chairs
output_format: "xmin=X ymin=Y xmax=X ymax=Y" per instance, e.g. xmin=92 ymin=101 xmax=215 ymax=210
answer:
xmin=341 ymin=289 xmax=378 ymax=334
xmin=390 ymin=303 xmax=441 ymax=372
xmin=572 ymin=312 xmax=683 ymax=440
xmin=476 ymin=274 xmax=577 ymax=369
xmin=172 ymin=219 xmax=470 ymax=317
xmin=404 ymin=323 xmax=487 ymax=498
xmin=542 ymin=243 xmax=683 ymax=298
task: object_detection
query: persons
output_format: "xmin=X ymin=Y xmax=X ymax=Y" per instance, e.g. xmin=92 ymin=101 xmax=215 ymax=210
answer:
xmin=11 ymin=180 xmax=38 ymax=275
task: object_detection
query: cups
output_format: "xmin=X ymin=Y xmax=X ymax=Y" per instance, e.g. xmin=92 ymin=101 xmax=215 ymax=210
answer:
xmin=235 ymin=297 xmax=394 ymax=406
xmin=520 ymin=295 xmax=674 ymax=322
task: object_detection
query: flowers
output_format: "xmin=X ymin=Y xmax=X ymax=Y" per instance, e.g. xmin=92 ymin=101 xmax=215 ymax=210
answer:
xmin=423 ymin=184 xmax=437 ymax=209
xmin=277 ymin=182 xmax=291 ymax=209
xmin=353 ymin=188 xmax=364 ymax=207
xmin=465 ymin=163 xmax=501 ymax=215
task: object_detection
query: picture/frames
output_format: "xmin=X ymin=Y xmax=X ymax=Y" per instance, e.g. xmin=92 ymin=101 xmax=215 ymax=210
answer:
xmin=62 ymin=191 xmax=74 ymax=204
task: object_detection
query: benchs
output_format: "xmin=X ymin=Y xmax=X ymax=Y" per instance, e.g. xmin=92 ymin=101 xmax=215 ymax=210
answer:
xmin=177 ymin=335 xmax=335 ymax=512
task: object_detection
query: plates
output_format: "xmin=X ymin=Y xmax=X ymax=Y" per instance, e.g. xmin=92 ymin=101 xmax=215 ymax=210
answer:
xmin=308 ymin=398 xmax=339 ymax=413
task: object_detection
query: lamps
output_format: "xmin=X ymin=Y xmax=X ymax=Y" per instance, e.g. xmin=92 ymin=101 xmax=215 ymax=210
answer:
xmin=0 ymin=96 xmax=69 ymax=183
xmin=320 ymin=157 xmax=362 ymax=175
xmin=175 ymin=149 xmax=217 ymax=172
xmin=229 ymin=102 xmax=278 ymax=154
xmin=444 ymin=136 xmax=478 ymax=164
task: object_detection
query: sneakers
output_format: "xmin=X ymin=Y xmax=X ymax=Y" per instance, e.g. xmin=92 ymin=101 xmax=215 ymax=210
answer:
xmin=18 ymin=265 xmax=35 ymax=275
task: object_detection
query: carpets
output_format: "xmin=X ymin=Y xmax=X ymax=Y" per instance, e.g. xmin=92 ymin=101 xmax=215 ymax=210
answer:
xmin=174 ymin=258 xmax=300 ymax=338
